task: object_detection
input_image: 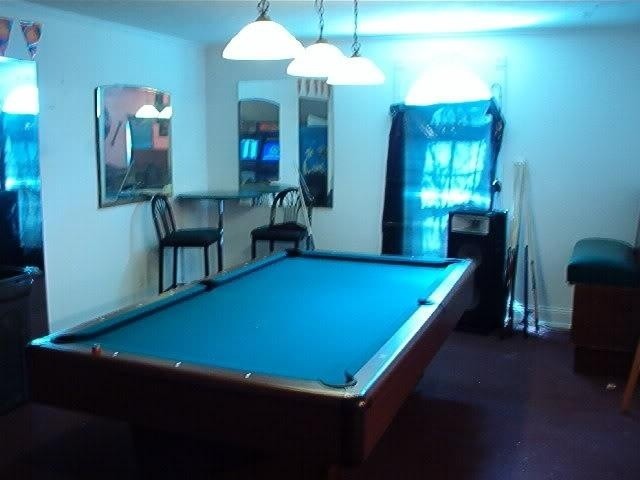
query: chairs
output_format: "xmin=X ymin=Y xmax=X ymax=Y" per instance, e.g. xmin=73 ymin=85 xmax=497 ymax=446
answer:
xmin=140 ymin=188 xmax=316 ymax=291
xmin=566 ymin=220 xmax=640 ymax=373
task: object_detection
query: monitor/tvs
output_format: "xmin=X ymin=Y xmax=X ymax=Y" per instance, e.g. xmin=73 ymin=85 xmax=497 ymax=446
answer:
xmin=242 ymin=139 xmax=257 ymax=161
xmin=260 ymin=139 xmax=281 ymax=162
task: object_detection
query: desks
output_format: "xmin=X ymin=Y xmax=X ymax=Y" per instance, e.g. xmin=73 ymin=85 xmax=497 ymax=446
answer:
xmin=20 ymin=245 xmax=481 ymax=480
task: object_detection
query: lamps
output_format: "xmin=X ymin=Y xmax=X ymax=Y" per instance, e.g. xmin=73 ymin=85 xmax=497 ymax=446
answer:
xmin=221 ymin=0 xmax=387 ymax=90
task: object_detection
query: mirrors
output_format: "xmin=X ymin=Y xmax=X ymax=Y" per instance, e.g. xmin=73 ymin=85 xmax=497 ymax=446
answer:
xmin=96 ymin=83 xmax=174 ymax=207
xmin=233 ymin=80 xmax=335 ymax=211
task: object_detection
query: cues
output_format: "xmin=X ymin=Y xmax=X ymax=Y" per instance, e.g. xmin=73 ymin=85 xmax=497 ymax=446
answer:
xmin=506 ymin=161 xmax=539 ymax=335
xmin=292 ymin=163 xmax=315 ymax=252
